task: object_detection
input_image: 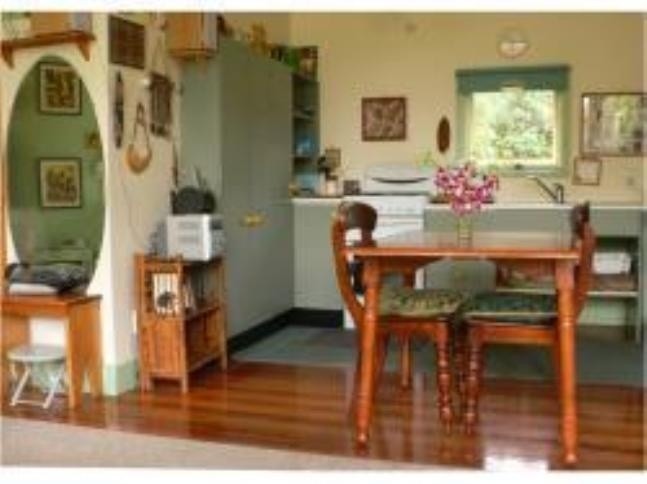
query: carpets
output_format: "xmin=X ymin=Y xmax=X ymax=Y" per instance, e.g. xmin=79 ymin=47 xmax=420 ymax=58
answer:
xmin=231 ymin=323 xmax=642 ymax=386
xmin=2 ymin=418 xmax=481 ymax=467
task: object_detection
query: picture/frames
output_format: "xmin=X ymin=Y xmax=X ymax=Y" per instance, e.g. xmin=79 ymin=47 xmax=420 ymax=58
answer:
xmin=360 ymin=98 xmax=406 ymax=142
xmin=37 ymin=62 xmax=82 ymax=116
xmin=580 ymin=91 xmax=647 ymax=157
xmin=36 ymin=156 xmax=83 ymax=209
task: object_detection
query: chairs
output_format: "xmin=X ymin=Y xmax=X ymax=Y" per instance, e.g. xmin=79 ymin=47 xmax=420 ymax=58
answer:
xmin=331 ymin=201 xmax=592 ymax=461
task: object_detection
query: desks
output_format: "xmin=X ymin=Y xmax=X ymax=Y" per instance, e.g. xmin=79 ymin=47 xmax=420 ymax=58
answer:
xmin=2 ymin=285 xmax=101 ymax=413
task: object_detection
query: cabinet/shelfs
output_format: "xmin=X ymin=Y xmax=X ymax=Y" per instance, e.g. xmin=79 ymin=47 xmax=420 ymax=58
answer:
xmin=135 ymin=252 xmax=226 ymax=396
xmin=178 ymin=38 xmax=320 ymax=340
xmin=560 ymin=211 xmax=642 ymax=347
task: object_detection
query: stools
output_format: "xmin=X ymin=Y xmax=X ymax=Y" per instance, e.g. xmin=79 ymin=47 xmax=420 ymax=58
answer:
xmin=3 ymin=344 xmax=70 ymax=409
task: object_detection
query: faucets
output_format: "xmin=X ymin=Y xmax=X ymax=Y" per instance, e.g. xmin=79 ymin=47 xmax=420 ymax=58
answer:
xmin=525 ymin=175 xmax=565 ymax=204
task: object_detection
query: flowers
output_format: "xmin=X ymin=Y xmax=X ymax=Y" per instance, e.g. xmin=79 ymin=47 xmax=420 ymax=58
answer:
xmin=434 ymin=163 xmax=500 ymax=218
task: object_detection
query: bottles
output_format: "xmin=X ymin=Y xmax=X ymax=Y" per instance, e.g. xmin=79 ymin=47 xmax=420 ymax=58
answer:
xmin=319 ymin=156 xmax=338 ymax=196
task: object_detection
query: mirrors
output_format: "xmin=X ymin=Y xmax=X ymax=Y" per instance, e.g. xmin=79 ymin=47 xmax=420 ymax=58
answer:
xmin=0 ymin=53 xmax=108 ymax=297
